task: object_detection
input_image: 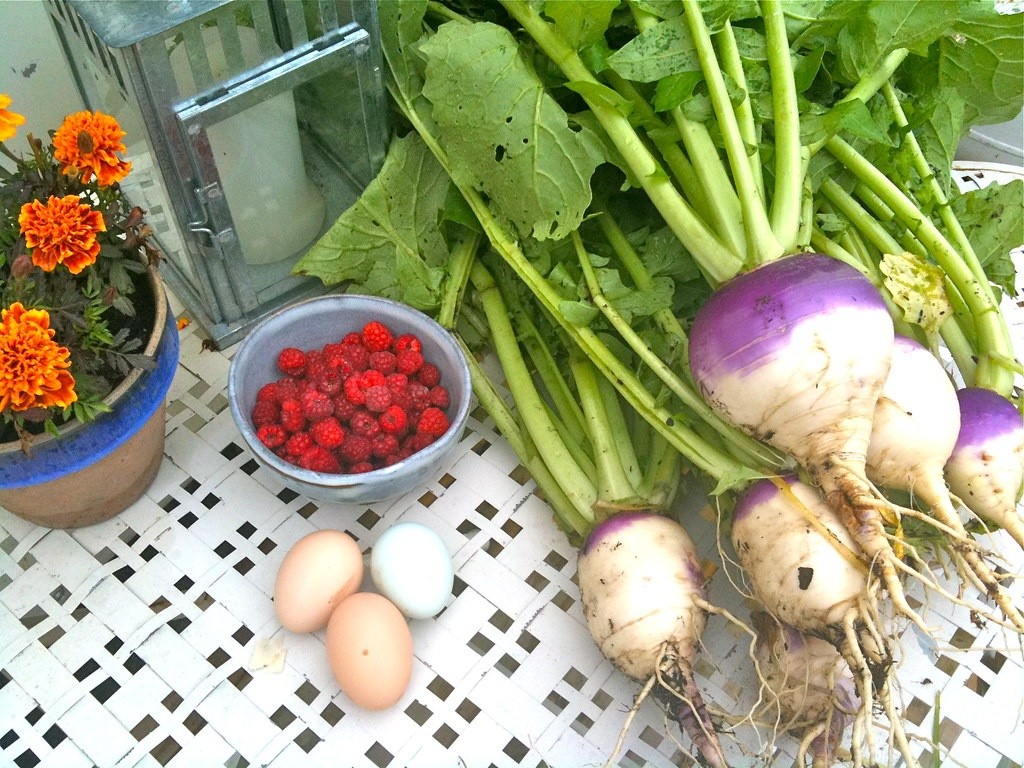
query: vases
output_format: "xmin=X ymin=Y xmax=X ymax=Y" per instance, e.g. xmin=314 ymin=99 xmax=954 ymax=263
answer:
xmin=2 ymin=231 xmax=180 ymax=532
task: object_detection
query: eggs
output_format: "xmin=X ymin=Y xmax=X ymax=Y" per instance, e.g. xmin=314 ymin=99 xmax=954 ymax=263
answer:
xmin=274 ymin=519 xmax=454 ymax=710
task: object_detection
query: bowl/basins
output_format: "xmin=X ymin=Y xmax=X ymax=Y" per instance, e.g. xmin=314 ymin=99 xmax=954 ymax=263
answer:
xmin=227 ymin=295 xmax=474 ymax=506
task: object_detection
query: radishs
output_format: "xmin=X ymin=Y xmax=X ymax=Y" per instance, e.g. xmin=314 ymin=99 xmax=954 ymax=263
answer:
xmin=580 ymin=261 xmax=1022 ymax=768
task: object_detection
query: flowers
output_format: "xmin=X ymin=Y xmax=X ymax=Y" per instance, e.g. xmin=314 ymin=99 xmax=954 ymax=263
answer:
xmin=0 ymin=97 xmax=169 ymax=459
